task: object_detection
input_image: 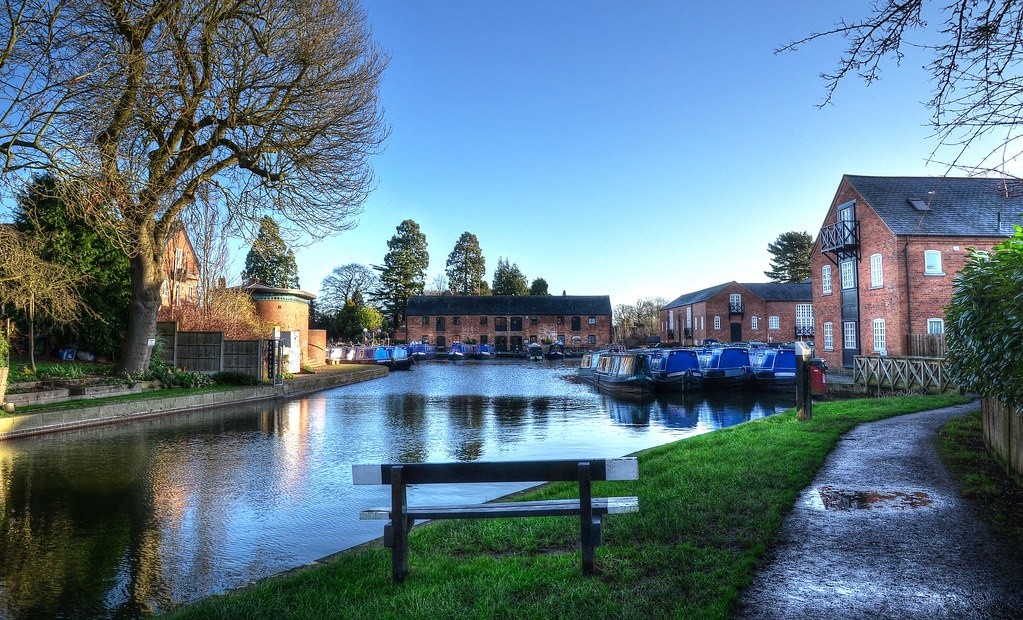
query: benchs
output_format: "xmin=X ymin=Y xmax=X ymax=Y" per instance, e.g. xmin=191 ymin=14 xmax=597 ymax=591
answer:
xmin=351 ymin=457 xmax=640 ymax=583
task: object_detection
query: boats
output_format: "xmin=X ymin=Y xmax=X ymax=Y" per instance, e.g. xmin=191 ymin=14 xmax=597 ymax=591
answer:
xmin=655 ymin=396 xmax=704 ymax=429
xmin=447 ymin=343 xmax=466 ymax=359
xmin=593 ymin=348 xmax=653 ymax=398
xmin=651 ymin=349 xmax=704 ymax=389
xmin=752 ymin=348 xmax=800 ymax=384
xmin=326 ymin=344 xmax=413 ymax=371
xmin=547 ymin=342 xmax=565 ymax=360
xmin=525 ymin=343 xmax=544 ymax=361
xmin=473 ymin=344 xmax=495 ymax=360
xmin=706 ymin=346 xmax=751 ymax=388
xmin=607 ymin=399 xmax=653 ymax=427
xmin=411 ymin=343 xmax=436 ymax=359
xmin=578 ymin=353 xmax=602 ymax=377
